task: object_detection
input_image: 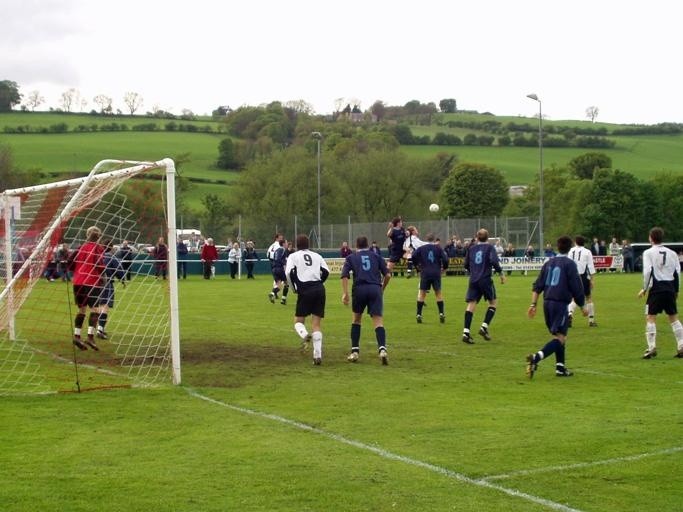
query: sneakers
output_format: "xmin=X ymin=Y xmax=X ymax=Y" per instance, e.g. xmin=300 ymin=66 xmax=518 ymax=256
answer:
xmin=72 ymin=327 xmax=108 ymax=351
xmin=268 ymin=290 xmax=491 ymax=363
xmin=526 ymin=311 xmax=683 ymax=379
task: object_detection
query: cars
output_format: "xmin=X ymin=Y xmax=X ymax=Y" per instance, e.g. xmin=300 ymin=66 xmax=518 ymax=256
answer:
xmin=213 ymin=244 xmax=226 ymax=250
xmin=112 ymin=243 xmax=155 ymax=253
xmin=53 ymin=243 xmax=81 ymax=253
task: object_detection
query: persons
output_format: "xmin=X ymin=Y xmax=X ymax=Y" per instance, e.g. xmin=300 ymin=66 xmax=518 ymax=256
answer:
xmin=368 ymin=242 xmax=381 ymax=253
xmin=152 ymin=235 xmax=262 ymax=279
xmin=491 ymin=237 xmax=504 ymax=275
xmin=591 ymin=237 xmax=633 ymax=273
xmin=525 ymin=245 xmax=535 ymax=257
xmin=462 ymin=228 xmax=506 ymax=344
xmin=636 ymin=226 xmax=682 ymax=361
xmin=342 ymin=236 xmax=390 ymax=366
xmin=284 ymin=234 xmax=330 ymax=365
xmin=502 ymin=241 xmax=516 ymax=275
xmin=340 ymin=242 xmax=350 ymax=257
xmin=44 ymin=226 xmax=134 ymax=351
xmin=433 ymin=233 xmax=476 ymax=275
xmin=566 ymin=236 xmax=595 ymax=328
xmin=266 ymin=233 xmax=295 ymax=306
xmin=543 ymin=243 xmax=558 ymax=257
xmin=385 ymin=215 xmax=428 ymax=278
xmin=413 ymin=234 xmax=449 ymax=323
xmin=525 ymin=238 xmax=588 ymax=380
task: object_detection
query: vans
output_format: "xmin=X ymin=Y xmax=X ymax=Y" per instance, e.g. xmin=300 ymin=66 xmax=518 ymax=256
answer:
xmin=176 ymin=228 xmax=205 ymax=252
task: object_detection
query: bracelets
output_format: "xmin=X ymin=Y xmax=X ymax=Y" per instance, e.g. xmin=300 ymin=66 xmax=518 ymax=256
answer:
xmin=529 ymin=302 xmax=537 ymax=307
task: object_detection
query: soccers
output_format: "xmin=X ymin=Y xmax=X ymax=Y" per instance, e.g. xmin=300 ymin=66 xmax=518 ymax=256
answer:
xmin=429 ymin=203 xmax=439 ymax=213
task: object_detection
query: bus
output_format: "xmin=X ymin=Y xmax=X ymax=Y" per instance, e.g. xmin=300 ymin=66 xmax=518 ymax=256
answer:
xmin=464 ymin=237 xmax=506 ymax=250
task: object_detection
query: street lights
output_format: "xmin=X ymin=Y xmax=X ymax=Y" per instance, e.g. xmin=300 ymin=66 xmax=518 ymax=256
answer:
xmin=527 ymin=91 xmax=546 ymax=263
xmin=309 ymin=130 xmax=321 ymax=245
